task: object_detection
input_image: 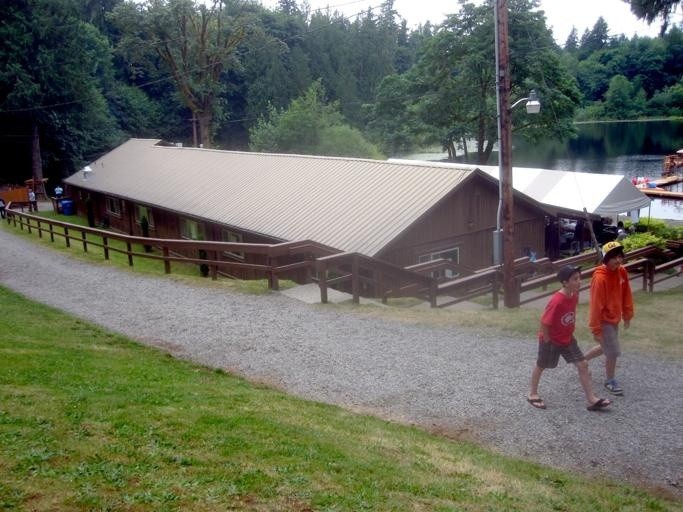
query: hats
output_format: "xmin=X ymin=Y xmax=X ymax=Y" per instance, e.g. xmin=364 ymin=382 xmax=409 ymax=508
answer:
xmin=557 ymin=265 xmax=583 ymax=282
xmin=601 ymin=241 xmax=624 ymax=264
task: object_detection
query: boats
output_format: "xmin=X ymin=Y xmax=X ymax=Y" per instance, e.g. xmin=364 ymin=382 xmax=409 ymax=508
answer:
xmin=671 ymin=149 xmax=683 ymax=168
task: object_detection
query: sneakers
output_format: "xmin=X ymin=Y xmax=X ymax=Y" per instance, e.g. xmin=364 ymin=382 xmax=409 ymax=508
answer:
xmin=603 ymin=379 xmax=624 ymax=395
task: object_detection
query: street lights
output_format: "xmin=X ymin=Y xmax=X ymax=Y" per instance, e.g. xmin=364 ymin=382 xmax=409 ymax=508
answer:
xmin=497 ymin=90 xmax=540 ymax=308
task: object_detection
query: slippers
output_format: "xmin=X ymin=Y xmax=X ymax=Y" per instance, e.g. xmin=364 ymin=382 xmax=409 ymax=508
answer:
xmin=587 ymin=398 xmax=613 ymax=410
xmin=527 ymin=398 xmax=546 ymax=409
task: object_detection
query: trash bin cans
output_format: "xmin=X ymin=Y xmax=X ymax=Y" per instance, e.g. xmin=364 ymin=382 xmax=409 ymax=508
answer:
xmin=61 ymin=200 xmax=73 ymax=216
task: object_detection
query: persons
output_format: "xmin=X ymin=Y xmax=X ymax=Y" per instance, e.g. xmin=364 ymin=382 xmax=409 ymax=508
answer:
xmin=583 ymin=241 xmax=634 ymax=397
xmin=526 ymin=265 xmax=612 ymax=412
xmin=0 ymin=186 xmax=64 ymax=219
xmin=546 ymin=220 xmax=636 ymax=254
